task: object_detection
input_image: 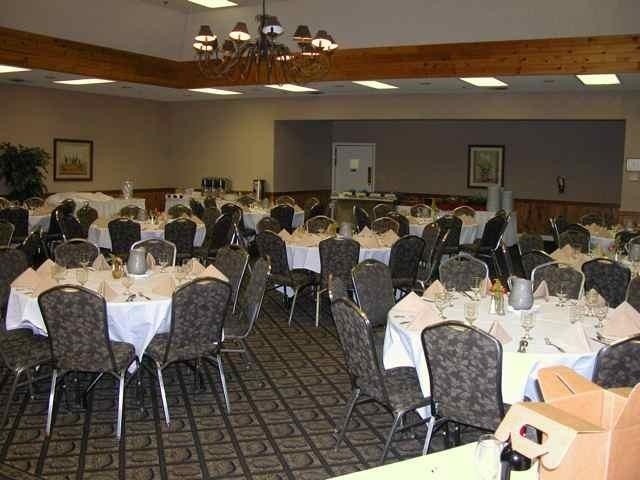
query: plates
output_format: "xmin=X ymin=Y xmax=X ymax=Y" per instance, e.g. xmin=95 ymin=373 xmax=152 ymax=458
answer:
xmin=486 ymin=184 xmax=514 ymax=213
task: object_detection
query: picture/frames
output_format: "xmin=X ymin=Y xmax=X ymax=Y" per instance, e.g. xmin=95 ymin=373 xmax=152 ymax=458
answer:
xmin=467 ymin=144 xmax=505 ymax=188
xmin=53 ymin=138 xmax=93 ymax=181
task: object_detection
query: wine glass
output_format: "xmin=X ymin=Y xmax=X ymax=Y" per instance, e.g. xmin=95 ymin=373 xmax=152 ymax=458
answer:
xmin=50 ymin=246 xmax=194 ymax=301
xmin=424 ymin=273 xmax=611 ymax=354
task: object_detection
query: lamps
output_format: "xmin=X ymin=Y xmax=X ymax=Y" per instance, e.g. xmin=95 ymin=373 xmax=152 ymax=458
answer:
xmin=189 ymin=0 xmax=340 ymax=87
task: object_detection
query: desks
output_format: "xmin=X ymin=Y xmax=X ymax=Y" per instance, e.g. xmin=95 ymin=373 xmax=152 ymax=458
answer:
xmin=318 ymin=436 xmax=538 ymax=479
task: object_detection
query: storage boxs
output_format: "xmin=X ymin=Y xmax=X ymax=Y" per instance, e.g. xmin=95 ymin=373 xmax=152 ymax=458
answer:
xmin=492 ymin=365 xmax=640 ymax=480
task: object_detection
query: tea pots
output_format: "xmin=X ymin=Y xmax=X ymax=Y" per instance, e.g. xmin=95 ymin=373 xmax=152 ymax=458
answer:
xmin=338 ymin=221 xmax=353 ymax=240
xmin=623 ymin=236 xmax=640 ymax=261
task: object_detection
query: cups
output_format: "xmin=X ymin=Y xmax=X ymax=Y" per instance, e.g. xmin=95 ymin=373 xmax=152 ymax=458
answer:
xmin=122 ymin=180 xmax=135 ymax=202
